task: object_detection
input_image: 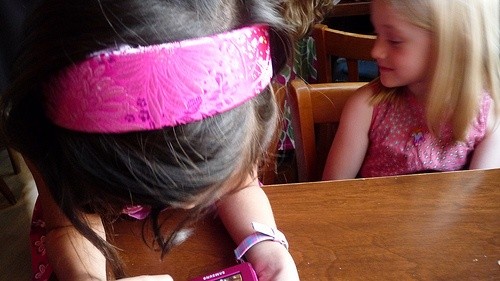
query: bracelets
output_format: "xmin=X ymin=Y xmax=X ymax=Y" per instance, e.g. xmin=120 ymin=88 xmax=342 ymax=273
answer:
xmin=234 ymin=223 xmax=288 ymax=265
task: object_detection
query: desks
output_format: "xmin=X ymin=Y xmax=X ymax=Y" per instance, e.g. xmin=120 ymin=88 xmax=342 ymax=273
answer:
xmin=105 ymin=167 xmax=500 ymax=281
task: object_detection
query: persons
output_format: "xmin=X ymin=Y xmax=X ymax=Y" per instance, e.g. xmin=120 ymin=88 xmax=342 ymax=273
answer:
xmin=324 ymin=0 xmax=500 ymax=179
xmin=0 ymin=0 xmax=339 ymax=281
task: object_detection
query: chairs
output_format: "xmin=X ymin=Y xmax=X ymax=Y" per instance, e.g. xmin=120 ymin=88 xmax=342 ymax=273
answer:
xmin=313 ymin=24 xmax=377 ymax=83
xmin=287 ymin=79 xmax=370 ymax=182
